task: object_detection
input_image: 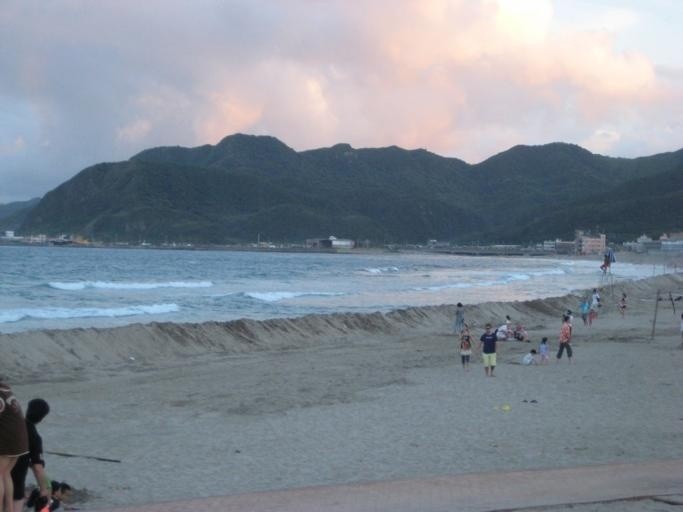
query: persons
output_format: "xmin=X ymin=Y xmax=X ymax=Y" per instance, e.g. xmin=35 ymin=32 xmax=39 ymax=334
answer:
xmin=0 ymin=382 xmax=74 ymax=512
xmin=452 ymin=289 xmax=627 ymax=379
xmin=599 ymin=254 xmax=608 ymax=274
